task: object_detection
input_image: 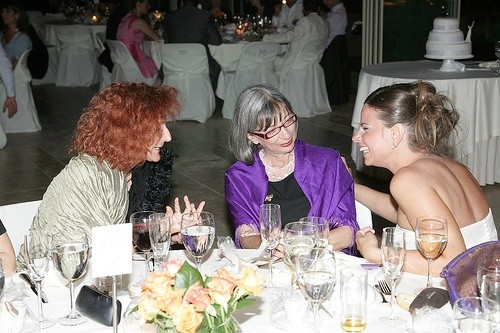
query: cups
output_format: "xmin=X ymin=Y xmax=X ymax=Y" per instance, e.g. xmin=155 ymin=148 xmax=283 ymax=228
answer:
xmin=338 ymin=267 xmax=368 ymax=333
xmin=451 ymin=297 xmax=500 ymax=333
xmin=99 ymin=275 xmax=122 ymax=287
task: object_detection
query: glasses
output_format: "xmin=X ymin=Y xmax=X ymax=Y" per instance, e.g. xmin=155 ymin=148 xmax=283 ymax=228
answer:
xmin=247 ymin=109 xmax=297 ymax=140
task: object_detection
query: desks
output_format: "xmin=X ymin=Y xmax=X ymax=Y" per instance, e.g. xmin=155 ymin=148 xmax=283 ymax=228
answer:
xmin=0 ymin=248 xmax=500 ymax=333
xmin=350 ymin=61 xmax=500 ymax=188
xmin=33 ymin=16 xmax=109 ymax=56
xmin=146 ymin=21 xmax=296 ymax=100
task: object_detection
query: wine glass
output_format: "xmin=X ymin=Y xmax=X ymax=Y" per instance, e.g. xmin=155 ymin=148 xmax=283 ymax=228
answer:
xmin=0 ymin=252 xmax=11 ymax=301
xmin=129 ymin=211 xmax=157 ymax=276
xmin=477 ymin=259 xmax=500 ymax=321
xmin=180 ymin=211 xmax=215 ymax=274
xmin=149 ymin=212 xmax=171 ymax=271
xmin=378 ymin=227 xmax=407 ymax=327
xmin=23 ymin=230 xmax=57 ymax=328
xmin=222 ymin=13 xmax=272 ymax=40
xmin=51 ymin=230 xmax=89 ymax=326
xmin=260 ymin=203 xmax=281 ymax=288
xmin=413 ymin=215 xmax=448 ymax=296
xmin=281 ymin=215 xmax=337 ymax=329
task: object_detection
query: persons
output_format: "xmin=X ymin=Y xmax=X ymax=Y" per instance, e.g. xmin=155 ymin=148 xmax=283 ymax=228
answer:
xmin=116 ymin=0 xmax=165 ymax=77
xmin=226 ymin=86 xmax=362 ymax=257
xmin=0 ymin=43 xmax=18 ymax=118
xmin=1 ymin=0 xmax=32 ymax=69
xmin=271 ymin=0 xmax=305 ymax=33
xmin=323 ymin=0 xmax=348 ymax=48
xmin=340 ymin=82 xmax=498 ymax=274
xmin=250 ymin=0 xmax=273 ymax=20
xmin=0 ymin=220 xmax=17 ymax=274
xmin=163 ymin=0 xmax=223 ymax=93
xmin=263 ymin=0 xmax=329 ymax=70
xmin=108 ymin=0 xmax=121 ymax=13
xmin=207 ymin=0 xmax=232 ymax=23
xmin=17 ymin=81 xmax=205 ymax=270
xmin=98 ymin=0 xmax=128 ymax=72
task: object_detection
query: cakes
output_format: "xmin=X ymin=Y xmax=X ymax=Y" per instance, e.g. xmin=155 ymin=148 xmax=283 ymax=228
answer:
xmin=426 ymin=17 xmax=472 ymax=57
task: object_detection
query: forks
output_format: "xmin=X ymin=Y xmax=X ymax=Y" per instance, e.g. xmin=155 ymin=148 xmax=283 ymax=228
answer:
xmin=378 ymin=280 xmax=396 ymax=298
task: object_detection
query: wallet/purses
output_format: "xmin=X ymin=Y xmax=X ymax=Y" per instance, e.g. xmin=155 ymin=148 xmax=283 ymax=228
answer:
xmin=75 ymin=285 xmax=122 ymax=327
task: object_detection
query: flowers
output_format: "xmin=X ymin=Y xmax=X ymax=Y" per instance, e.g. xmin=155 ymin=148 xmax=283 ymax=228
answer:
xmin=122 ymin=257 xmax=266 ymax=333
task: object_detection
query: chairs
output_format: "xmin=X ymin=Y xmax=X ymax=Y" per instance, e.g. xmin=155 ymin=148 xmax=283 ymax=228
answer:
xmin=0 ymin=10 xmax=334 ymax=134
xmin=0 ymin=197 xmax=45 ymax=232
